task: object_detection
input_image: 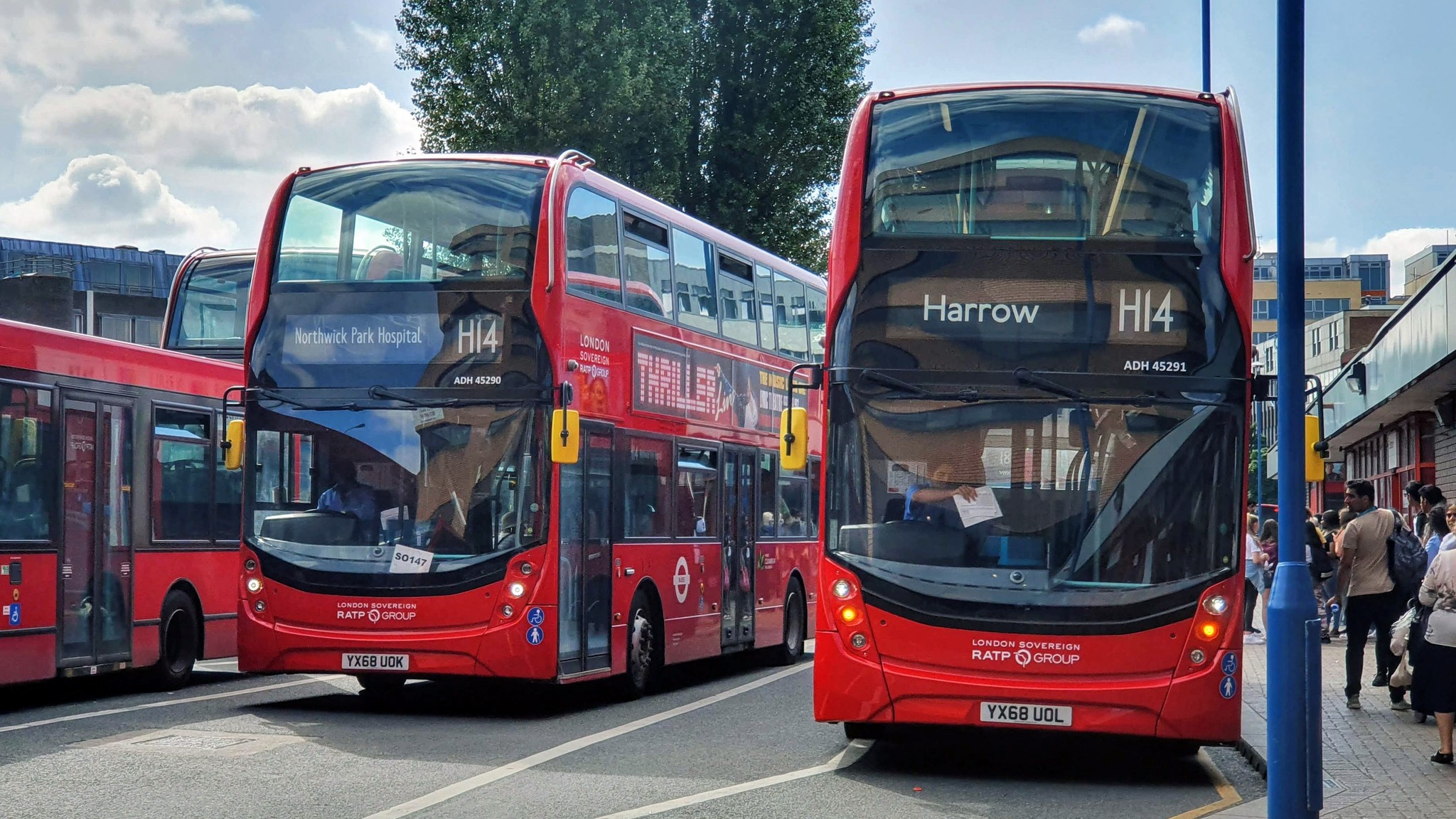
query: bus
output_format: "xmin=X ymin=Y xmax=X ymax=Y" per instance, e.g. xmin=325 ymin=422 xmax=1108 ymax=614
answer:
xmin=1 ymin=315 xmax=311 ymax=687
xmin=773 ymin=82 xmax=1332 ymax=745
xmin=213 ymin=145 xmax=834 ymax=691
xmin=159 ymin=243 xmax=477 ymax=358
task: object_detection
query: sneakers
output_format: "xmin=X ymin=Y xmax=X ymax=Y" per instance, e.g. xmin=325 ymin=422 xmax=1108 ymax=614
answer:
xmin=1389 ymin=699 xmax=1412 ymax=710
xmin=1372 ymin=672 xmax=1388 ymax=686
xmin=1346 ymin=693 xmax=1360 ymax=708
xmin=1431 ymin=747 xmax=1453 ymax=762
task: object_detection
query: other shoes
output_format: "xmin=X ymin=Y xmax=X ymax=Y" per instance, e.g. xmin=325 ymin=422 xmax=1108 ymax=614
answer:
xmin=1340 ymin=633 xmax=1348 ymax=640
xmin=1327 ymin=630 xmax=1340 ymax=637
xmin=1331 ymin=617 xmax=1334 ymax=622
xmin=1244 ymin=633 xmax=1265 ymax=644
xmin=1250 ymin=631 xmax=1264 ymax=637
xmin=1320 ymin=630 xmax=1331 ymax=643
xmin=1367 ymin=628 xmax=1377 ymax=638
xmin=1251 ymin=627 xmax=1261 ymax=634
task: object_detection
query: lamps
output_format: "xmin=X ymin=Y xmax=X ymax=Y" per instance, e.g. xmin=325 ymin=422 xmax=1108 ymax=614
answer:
xmin=1339 ymin=445 xmax=1344 ymax=451
xmin=1347 ymin=363 xmax=1366 ymax=396
xmin=1378 ymin=423 xmax=1387 ymax=431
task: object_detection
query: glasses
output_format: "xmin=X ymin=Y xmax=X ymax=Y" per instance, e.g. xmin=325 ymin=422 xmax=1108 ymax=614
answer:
xmin=1404 ymin=491 xmax=1409 ymax=497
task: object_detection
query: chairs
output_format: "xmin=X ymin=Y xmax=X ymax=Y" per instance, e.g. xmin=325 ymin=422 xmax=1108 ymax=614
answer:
xmin=0 ymin=457 xmax=240 ymax=541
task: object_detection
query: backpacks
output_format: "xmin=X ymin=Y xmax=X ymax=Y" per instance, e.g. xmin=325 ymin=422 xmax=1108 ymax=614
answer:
xmin=1386 ymin=508 xmax=1428 ymax=587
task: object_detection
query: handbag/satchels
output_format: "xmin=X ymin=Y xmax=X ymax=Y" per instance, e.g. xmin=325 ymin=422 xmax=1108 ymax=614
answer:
xmin=1311 ymin=548 xmax=1333 ymax=581
xmin=1390 ymin=606 xmax=1417 ymax=656
xmin=1389 ymin=645 xmax=1414 ymax=687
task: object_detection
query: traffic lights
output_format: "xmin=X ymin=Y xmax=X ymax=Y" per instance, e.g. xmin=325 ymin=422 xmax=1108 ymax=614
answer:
xmin=1254 ymin=504 xmax=1280 ymax=529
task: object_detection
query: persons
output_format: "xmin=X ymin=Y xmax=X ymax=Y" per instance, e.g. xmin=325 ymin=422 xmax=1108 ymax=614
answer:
xmin=318 ymin=460 xmax=376 ymax=522
xmin=1373 ymin=480 xmax=1456 ymax=763
xmin=904 ymin=457 xmax=978 ymax=524
xmin=1327 ymin=478 xmax=1411 ymax=711
xmin=1243 ymin=498 xmax=1279 ymax=644
xmin=492 ymin=510 xmax=527 ymax=550
xmin=749 ymin=468 xmax=793 ymax=528
xmin=1304 ymin=507 xmax=1376 ymax=644
